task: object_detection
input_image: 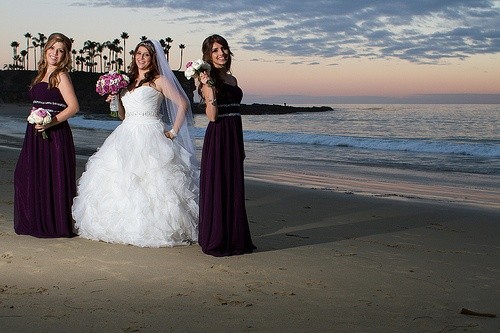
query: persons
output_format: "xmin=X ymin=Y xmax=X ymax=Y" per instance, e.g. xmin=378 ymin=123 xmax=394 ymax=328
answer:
xmin=13 ymin=33 xmax=80 ymax=239
xmin=72 ymin=39 xmax=200 ymax=248
xmin=195 ymin=34 xmax=258 ymax=256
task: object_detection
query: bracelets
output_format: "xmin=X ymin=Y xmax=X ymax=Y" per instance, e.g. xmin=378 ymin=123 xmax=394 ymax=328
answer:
xmin=170 ymin=128 xmax=177 ymax=137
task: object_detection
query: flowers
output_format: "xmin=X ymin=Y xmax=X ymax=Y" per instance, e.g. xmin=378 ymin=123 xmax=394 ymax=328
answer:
xmin=94 ymin=70 xmax=131 ymax=96
xmin=184 ymin=58 xmax=213 ymax=86
xmin=26 ymin=108 xmax=52 ymax=126
